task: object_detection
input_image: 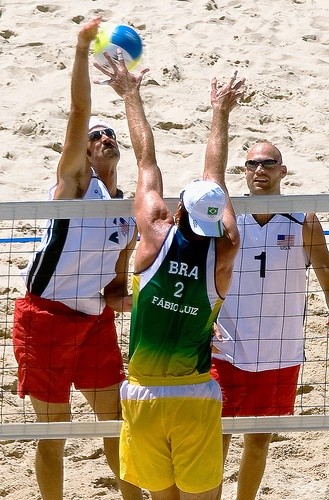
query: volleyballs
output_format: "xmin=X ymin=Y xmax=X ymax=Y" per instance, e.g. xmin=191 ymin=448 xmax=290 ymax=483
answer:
xmin=93 ymin=24 xmax=142 ymax=73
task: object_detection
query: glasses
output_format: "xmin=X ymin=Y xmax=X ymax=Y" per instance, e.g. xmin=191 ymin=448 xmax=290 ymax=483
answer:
xmin=88 ymin=128 xmax=116 ymax=139
xmin=245 ymin=160 xmax=281 ymax=169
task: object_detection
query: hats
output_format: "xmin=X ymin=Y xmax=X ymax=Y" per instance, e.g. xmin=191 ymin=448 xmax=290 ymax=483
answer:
xmin=183 ymin=181 xmax=226 ymax=237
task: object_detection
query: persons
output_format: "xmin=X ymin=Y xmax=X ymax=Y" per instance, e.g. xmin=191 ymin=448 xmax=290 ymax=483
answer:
xmin=212 ymin=142 xmax=329 ymax=500
xmin=13 ymin=14 xmax=143 ymax=499
xmin=92 ymin=46 xmax=247 ymax=500
